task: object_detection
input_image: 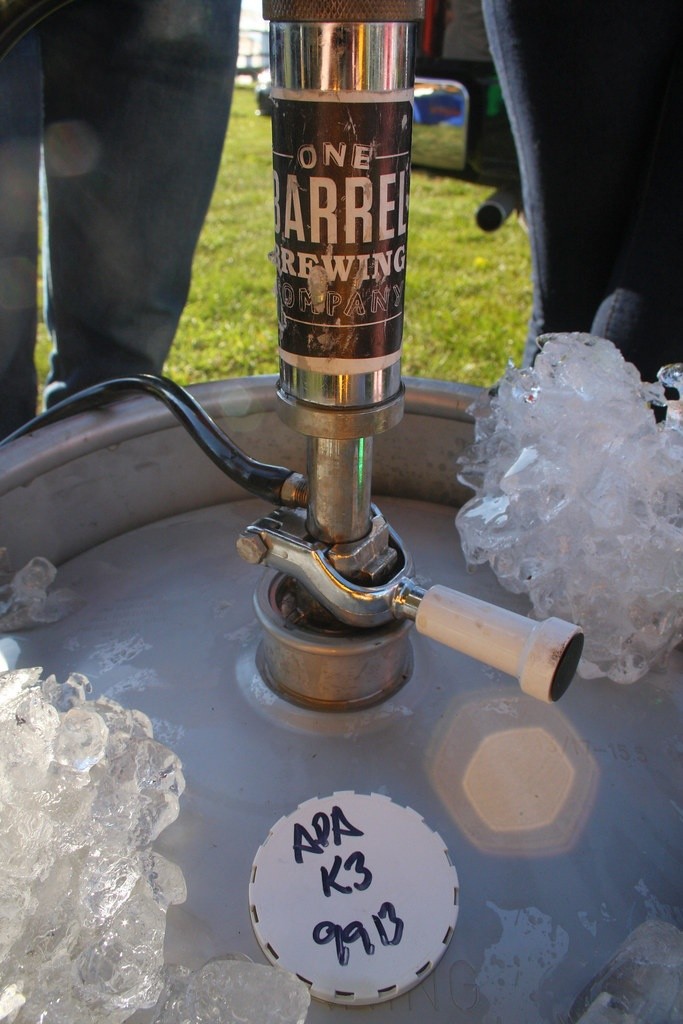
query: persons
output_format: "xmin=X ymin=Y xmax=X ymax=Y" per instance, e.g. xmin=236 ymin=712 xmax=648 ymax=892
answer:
xmin=1 ymin=0 xmax=241 ymax=442
xmin=475 ymin=0 xmax=682 ymax=418
